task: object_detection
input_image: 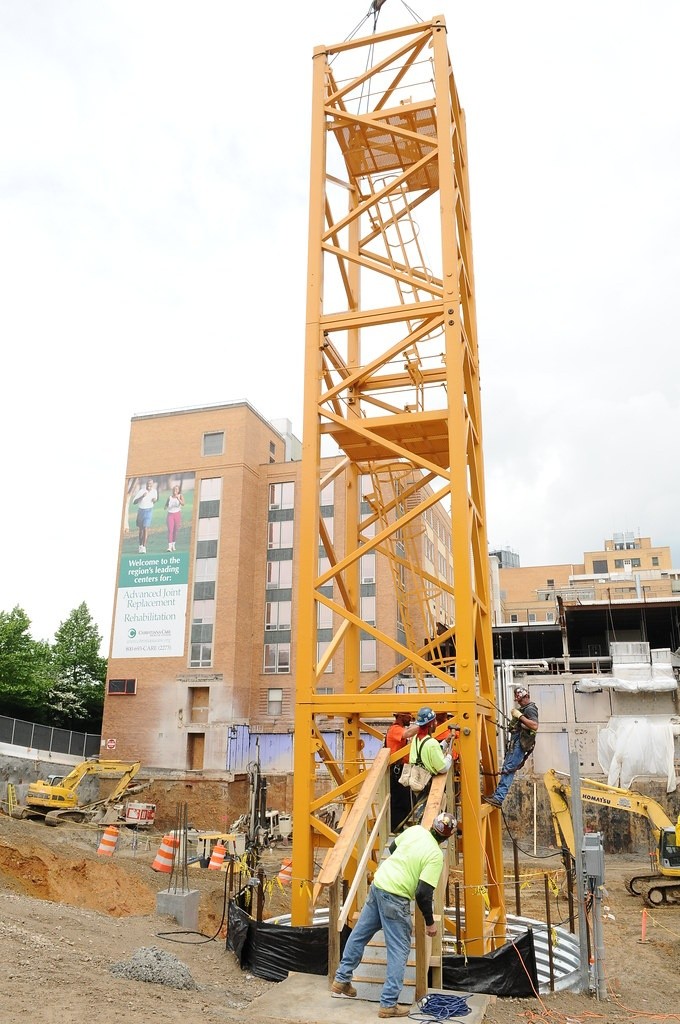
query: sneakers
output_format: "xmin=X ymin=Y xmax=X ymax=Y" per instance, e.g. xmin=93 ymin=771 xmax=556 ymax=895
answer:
xmin=331 ymin=980 xmax=357 ymax=997
xmin=378 ymin=1003 xmax=410 ymax=1018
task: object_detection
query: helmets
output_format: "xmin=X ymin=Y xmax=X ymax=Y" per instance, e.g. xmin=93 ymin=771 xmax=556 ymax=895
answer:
xmin=514 ymin=687 xmax=529 ymax=701
xmin=415 ymin=707 xmax=436 ymax=725
xmin=394 ymin=711 xmax=412 ymax=716
xmin=433 ymin=813 xmax=457 ymax=836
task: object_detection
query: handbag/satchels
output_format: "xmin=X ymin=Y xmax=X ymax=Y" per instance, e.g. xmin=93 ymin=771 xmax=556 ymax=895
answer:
xmin=398 ymin=737 xmax=432 ymax=798
xmin=520 ymin=730 xmax=534 ymax=751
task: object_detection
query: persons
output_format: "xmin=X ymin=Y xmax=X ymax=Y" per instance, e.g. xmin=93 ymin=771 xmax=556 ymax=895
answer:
xmin=385 ymin=707 xmax=460 ymax=833
xmin=331 ymin=812 xmax=457 ymax=1018
xmin=482 ymin=687 xmax=539 ymax=808
xmin=164 ymin=485 xmax=185 ymax=552
xmin=133 ymin=479 xmax=157 ymax=554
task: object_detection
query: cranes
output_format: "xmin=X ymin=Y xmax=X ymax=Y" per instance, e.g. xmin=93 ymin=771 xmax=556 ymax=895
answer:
xmin=286 ymin=0 xmax=512 ymax=1000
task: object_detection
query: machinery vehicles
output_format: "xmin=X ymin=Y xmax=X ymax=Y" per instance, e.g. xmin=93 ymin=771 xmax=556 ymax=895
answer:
xmin=543 ymin=768 xmax=680 ymax=912
xmin=10 ymin=756 xmax=154 ymax=829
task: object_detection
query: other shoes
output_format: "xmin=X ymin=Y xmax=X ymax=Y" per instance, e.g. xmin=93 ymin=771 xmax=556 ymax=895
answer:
xmin=481 ymin=795 xmax=502 ymax=809
